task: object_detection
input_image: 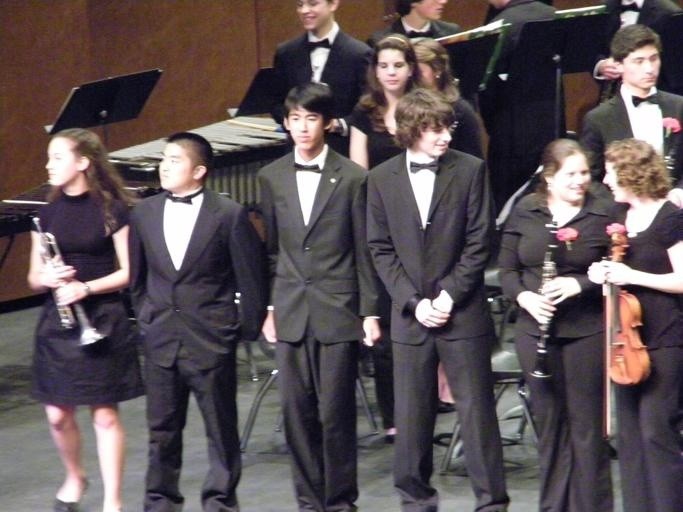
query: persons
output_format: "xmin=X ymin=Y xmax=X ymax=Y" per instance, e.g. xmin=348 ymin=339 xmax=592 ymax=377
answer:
xmin=365 ymin=91 xmax=512 ymax=512
xmin=259 ymin=83 xmax=383 ymax=511
xmin=123 ymin=131 xmax=268 ymax=511
xmin=499 ymin=138 xmax=625 ymax=512
xmin=410 ymin=36 xmax=488 ymax=415
xmin=588 ymin=0 xmax=683 ymax=102
xmin=28 ymin=129 xmax=149 ymax=512
xmin=579 ymin=24 xmax=683 ymax=208
xmin=364 ymin=0 xmax=568 ymax=211
xmin=350 ymin=34 xmax=423 ymax=440
xmin=268 ymin=1 xmax=370 ymax=155
xmin=586 ymin=137 xmax=683 ymax=511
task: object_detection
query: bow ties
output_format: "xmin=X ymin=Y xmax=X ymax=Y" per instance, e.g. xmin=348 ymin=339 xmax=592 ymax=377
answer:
xmin=303 ymin=38 xmax=330 ymax=51
xmin=632 ymin=93 xmax=658 ymax=106
xmin=409 ymin=29 xmax=433 ymax=38
xmin=167 ymin=193 xmax=193 ymax=205
xmin=618 ymin=3 xmax=639 ymax=14
xmin=409 ymin=160 xmax=440 ymax=175
xmin=293 ymin=160 xmax=321 ymax=174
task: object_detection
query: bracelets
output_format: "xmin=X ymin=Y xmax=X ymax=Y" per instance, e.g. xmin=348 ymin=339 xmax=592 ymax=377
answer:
xmin=84 ymin=282 xmax=91 ymax=295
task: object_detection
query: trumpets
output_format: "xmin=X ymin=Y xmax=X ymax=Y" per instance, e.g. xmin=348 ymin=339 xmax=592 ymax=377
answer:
xmin=33 ymin=217 xmax=107 ymax=350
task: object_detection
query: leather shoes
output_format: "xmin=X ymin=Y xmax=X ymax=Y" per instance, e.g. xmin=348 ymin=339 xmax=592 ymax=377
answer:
xmin=385 ymin=432 xmax=397 ymax=444
xmin=438 ymin=400 xmax=455 ymax=413
xmin=52 ymin=475 xmax=88 ymax=511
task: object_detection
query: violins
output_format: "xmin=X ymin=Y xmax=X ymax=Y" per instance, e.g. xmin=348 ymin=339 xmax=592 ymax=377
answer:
xmin=607 ymin=234 xmax=650 ymax=384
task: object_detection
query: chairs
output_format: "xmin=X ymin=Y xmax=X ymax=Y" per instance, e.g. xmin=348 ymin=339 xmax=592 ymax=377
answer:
xmin=484 ymin=284 xmax=507 ymax=340
xmin=239 ymin=332 xmax=380 ymax=454
xmin=439 ymin=348 xmax=544 ymax=477
